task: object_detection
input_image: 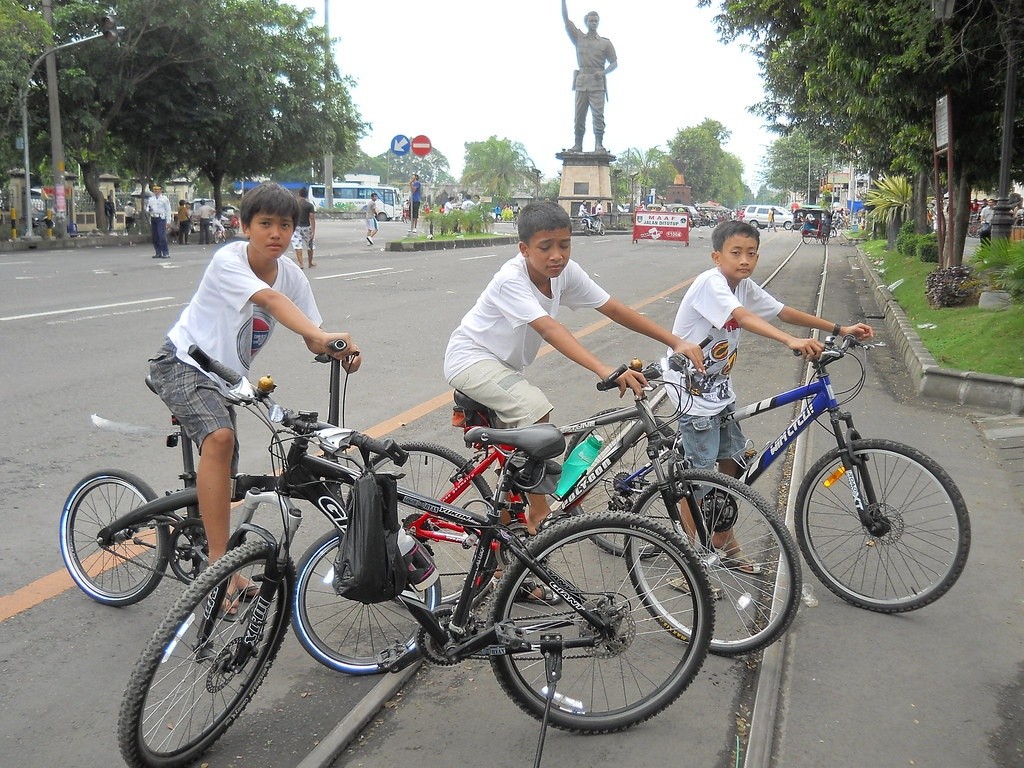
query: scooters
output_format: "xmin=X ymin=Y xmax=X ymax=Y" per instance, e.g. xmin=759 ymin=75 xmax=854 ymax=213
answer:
xmin=689 ymin=208 xmax=854 ymax=244
xmin=580 ymin=214 xmax=605 ymax=237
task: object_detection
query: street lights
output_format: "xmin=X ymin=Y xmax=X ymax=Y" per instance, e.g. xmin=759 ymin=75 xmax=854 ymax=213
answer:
xmin=630 ymin=172 xmax=639 ymax=213
xmin=22 ymin=26 xmax=127 ymax=250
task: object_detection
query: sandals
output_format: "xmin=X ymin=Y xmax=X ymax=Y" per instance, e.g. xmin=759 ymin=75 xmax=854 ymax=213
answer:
xmin=718 ymin=548 xmax=763 ymax=574
xmin=667 ymin=576 xmax=726 ymax=600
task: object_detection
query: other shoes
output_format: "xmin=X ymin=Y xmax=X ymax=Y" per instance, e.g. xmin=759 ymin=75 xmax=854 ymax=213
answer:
xmin=161 ymin=254 xmax=170 ymax=258
xmin=412 ymin=228 xmax=417 ymax=233
xmin=367 ymin=236 xmax=374 ymax=246
xmin=152 ymin=254 xmax=161 ymax=258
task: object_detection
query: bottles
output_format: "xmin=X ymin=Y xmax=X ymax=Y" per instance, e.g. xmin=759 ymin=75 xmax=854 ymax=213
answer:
xmin=554 ymin=435 xmax=604 ymax=498
xmin=397 ymin=526 xmax=439 ymax=591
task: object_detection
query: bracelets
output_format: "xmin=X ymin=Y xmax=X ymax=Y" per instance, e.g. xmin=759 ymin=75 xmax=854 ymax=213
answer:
xmin=832 ymin=323 xmax=841 ymax=336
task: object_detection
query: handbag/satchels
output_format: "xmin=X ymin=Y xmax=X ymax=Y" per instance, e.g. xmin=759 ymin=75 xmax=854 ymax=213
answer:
xmin=332 ymin=463 xmax=408 ymax=605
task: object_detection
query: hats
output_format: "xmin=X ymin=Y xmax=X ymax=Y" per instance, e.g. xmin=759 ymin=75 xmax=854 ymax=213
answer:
xmin=770 ymin=207 xmax=775 ymax=210
xmin=152 ymin=180 xmax=163 ymax=189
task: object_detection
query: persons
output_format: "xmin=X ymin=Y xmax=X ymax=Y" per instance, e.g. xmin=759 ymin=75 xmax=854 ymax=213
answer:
xmin=290 ymin=187 xmax=317 ymax=269
xmin=403 ymin=174 xmax=421 ymax=232
xmin=198 ymin=199 xmax=216 ymax=244
xmin=124 ymin=201 xmax=135 ymax=231
xmin=638 ymin=201 xmax=684 ymax=212
xmin=767 ymin=207 xmax=778 ymax=232
xmin=596 ymin=199 xmax=603 ymax=214
xmin=697 ymin=209 xmax=745 ymax=221
xmin=1014 ymin=203 xmax=1024 ymax=226
xmin=170 ymin=200 xmax=194 ymax=245
xmin=495 ymin=202 xmax=521 ymax=220
xmin=440 ymin=194 xmax=482 ymax=215
xmin=106 ymin=195 xmax=115 ymax=230
xmin=578 ymin=200 xmax=588 ymax=216
xmin=146 ymin=184 xmax=171 ymax=258
xmin=444 ymin=201 xmax=707 ymax=604
xmin=970 ymin=198 xmax=995 ymax=229
xmin=561 ymin=0 xmax=617 ymax=151
xmin=148 ymin=181 xmax=361 ymax=622
xmin=662 ymin=221 xmax=874 ymax=599
xmin=366 ymin=193 xmax=379 ymax=245
xmin=211 ymin=208 xmax=229 ymax=243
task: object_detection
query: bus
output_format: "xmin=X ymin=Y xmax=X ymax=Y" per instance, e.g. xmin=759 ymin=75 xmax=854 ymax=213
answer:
xmin=307 ymin=180 xmax=404 ymax=222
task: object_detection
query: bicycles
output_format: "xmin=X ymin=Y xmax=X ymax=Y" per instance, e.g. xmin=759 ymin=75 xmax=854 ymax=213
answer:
xmin=112 ymin=343 xmax=720 ymax=768
xmin=556 ymin=329 xmax=973 ymax=617
xmin=55 ymin=336 xmax=441 ymax=674
xmin=345 ymin=358 xmax=802 ymax=656
xmin=213 ymin=225 xmax=228 ymax=244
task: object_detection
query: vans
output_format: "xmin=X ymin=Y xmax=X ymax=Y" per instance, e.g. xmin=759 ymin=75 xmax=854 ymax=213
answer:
xmin=743 ymin=205 xmax=795 ymax=230
xmin=647 ymin=204 xmax=700 ymax=228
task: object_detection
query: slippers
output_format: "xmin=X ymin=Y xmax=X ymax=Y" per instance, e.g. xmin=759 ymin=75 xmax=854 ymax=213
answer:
xmin=514 ymin=583 xmax=563 ymax=605
xmin=526 ymin=509 xmax=587 ymax=544
xmin=202 ymin=590 xmax=239 ymax=621
xmin=239 ymin=581 xmax=261 ymax=603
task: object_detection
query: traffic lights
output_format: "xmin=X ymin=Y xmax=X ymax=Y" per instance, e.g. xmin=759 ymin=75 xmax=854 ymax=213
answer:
xmin=100 ymin=14 xmax=117 ymax=42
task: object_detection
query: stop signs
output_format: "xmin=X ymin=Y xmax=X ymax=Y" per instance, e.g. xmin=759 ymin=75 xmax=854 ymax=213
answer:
xmin=411 ymin=134 xmax=432 ymax=158
